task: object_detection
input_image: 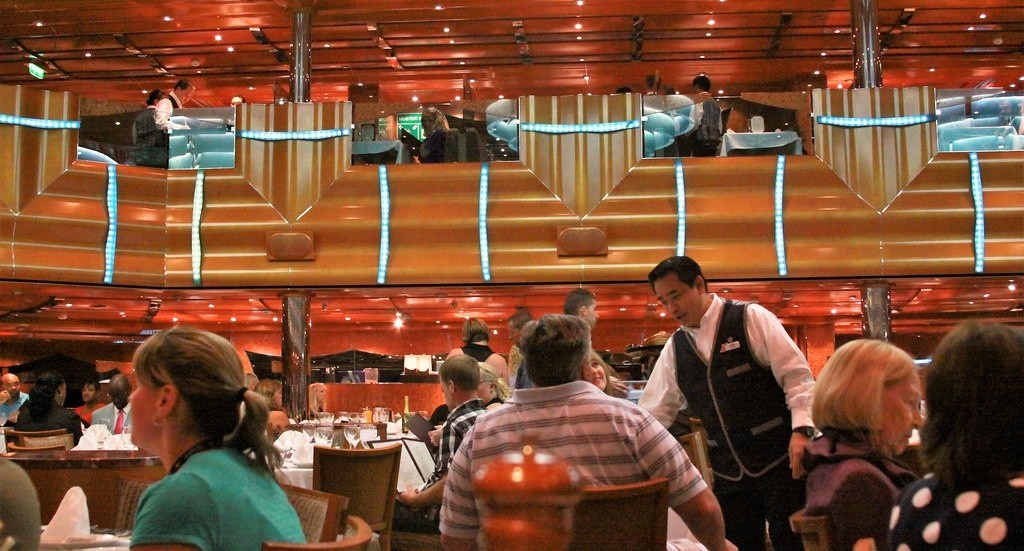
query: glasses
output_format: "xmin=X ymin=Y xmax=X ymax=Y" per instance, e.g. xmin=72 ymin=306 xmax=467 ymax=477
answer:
xmin=106 ymin=393 xmax=126 ymax=401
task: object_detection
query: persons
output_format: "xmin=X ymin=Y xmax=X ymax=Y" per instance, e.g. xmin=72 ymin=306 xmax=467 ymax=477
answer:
xmin=391 ymin=355 xmax=512 ymax=534
xmin=618 ymin=74 xmax=724 ymax=157
xmin=419 ymin=106 xmax=451 ymax=163
xmin=152 ymin=79 xmax=196 ymax=169
xmin=590 ymin=347 xmax=628 ymax=399
xmin=637 ymin=256 xmax=816 ymax=551
xmin=13 ymin=371 xmax=84 ymax=446
xmin=0 ymin=373 xmax=29 ymax=426
xmin=74 ymin=379 xmax=107 ymax=425
xmin=802 ymin=339 xmax=925 ymax=551
xmin=887 ymin=318 xmax=1024 ymax=551
xmin=222 ymin=94 xmax=245 ymax=130
xmin=563 ymin=288 xmax=600 ymax=328
xmin=90 ymin=374 xmax=132 ymax=435
xmin=128 ymin=326 xmax=308 ymax=551
xmin=438 ymin=314 xmax=739 ymax=551
xmin=445 ymin=317 xmax=509 ymax=387
xmin=506 ymin=313 xmax=539 ymax=389
xmin=994 ymin=99 xmax=1024 ymax=151
xmin=132 ymin=90 xmax=166 ymax=166
xmin=247 ymin=373 xmax=327 ymax=441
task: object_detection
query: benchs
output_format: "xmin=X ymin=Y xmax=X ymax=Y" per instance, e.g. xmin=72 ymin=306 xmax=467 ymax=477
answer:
xmin=439 ymin=128 xmax=490 ymax=162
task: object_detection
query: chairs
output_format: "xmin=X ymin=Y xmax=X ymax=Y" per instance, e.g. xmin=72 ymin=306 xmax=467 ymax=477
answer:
xmin=0 ymin=427 xmax=402 ymax=551
xmin=571 ymin=476 xmax=670 ymax=550
xmin=790 ymin=508 xmax=833 ymax=550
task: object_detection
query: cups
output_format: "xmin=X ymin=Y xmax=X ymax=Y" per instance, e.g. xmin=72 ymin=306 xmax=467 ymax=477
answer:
xmin=121 ymin=425 xmax=136 ymax=450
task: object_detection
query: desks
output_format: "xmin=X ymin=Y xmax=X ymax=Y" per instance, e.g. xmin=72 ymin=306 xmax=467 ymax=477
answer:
xmin=352 ymin=139 xmax=410 ymax=164
xmin=716 ymin=128 xmax=802 ymax=158
xmin=276 ymin=431 xmax=417 ymax=489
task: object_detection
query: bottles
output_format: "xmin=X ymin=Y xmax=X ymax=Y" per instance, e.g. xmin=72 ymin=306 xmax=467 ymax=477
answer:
xmin=402 ymin=395 xmax=410 ymax=434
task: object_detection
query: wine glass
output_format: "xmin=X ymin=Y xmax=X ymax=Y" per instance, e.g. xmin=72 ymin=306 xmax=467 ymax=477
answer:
xmin=302 ymin=406 xmax=390 ymax=450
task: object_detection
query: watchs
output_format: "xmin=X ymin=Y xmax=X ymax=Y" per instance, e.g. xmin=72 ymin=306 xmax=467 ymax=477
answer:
xmin=794 ymin=428 xmax=814 ymax=437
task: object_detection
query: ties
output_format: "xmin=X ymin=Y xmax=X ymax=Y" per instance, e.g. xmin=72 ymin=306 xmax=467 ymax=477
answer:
xmin=115 ymin=410 xmax=124 ymax=435
